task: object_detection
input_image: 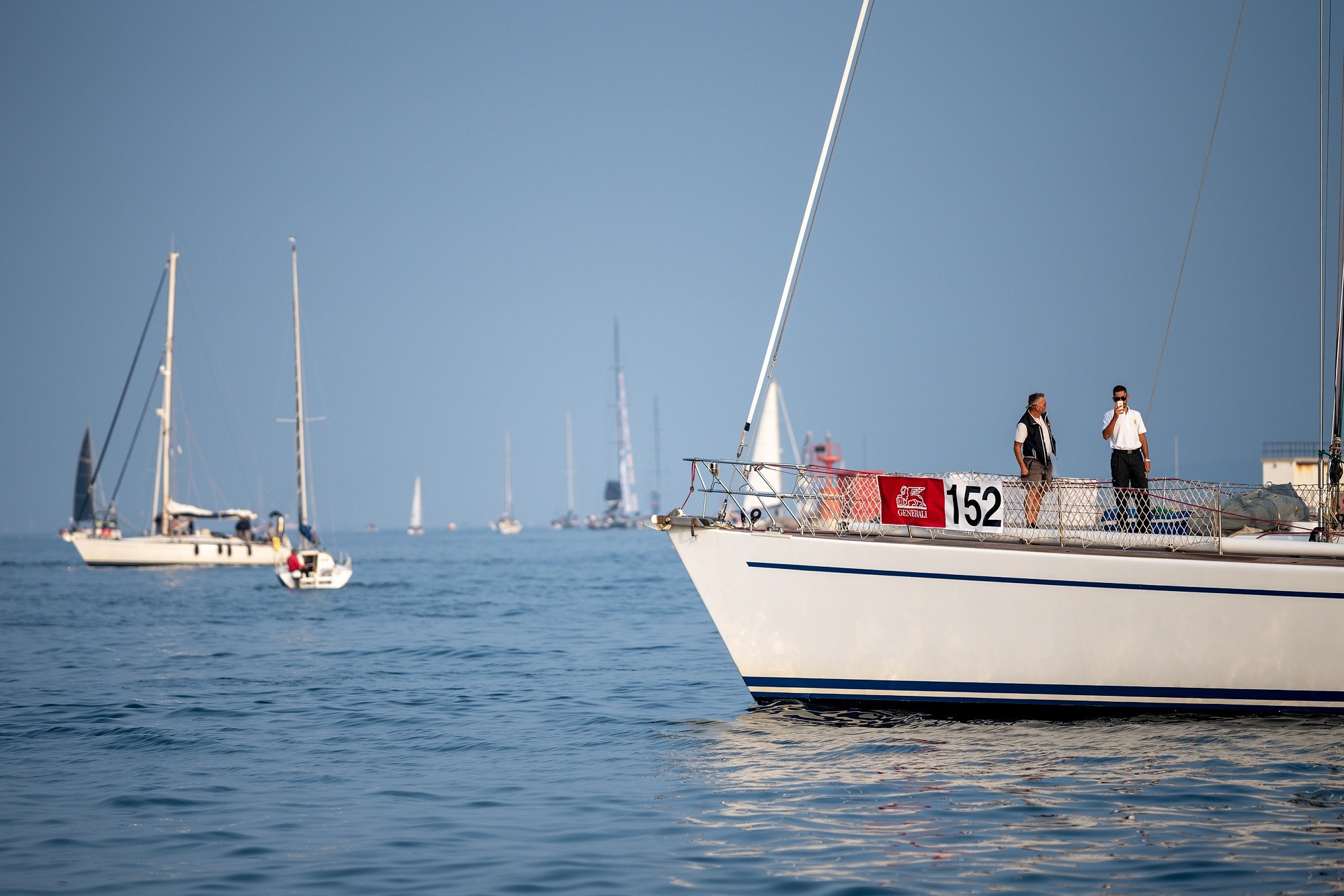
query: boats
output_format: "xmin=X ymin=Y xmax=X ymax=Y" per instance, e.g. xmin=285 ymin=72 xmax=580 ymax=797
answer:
xmin=652 ymin=0 xmax=1344 ymax=708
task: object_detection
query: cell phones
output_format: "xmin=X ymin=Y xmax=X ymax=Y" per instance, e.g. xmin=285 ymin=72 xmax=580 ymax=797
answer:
xmin=1117 ymin=400 xmax=1124 ymax=414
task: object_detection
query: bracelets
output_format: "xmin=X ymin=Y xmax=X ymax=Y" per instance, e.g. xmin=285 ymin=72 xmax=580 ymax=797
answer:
xmin=1144 ymin=459 xmax=1151 ymax=462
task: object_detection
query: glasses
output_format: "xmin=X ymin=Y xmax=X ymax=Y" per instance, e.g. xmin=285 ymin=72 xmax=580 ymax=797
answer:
xmin=1026 ymin=402 xmax=1047 ymax=409
xmin=1113 ymin=396 xmax=1127 ymax=401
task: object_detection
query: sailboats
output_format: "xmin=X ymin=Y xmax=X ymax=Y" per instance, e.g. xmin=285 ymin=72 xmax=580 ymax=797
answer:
xmin=274 ymin=237 xmax=355 ymax=593
xmin=588 ymin=324 xmax=642 ymax=531
xmin=59 ymin=253 xmax=292 ymax=569
xmin=551 ymin=409 xmax=580 ymax=531
xmin=497 ymin=431 xmax=522 ymax=534
xmin=408 ymin=476 xmax=425 ymax=535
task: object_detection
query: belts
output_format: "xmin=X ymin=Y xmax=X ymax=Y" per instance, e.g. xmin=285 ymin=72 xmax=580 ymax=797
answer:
xmin=1113 ymin=449 xmax=1140 ymax=454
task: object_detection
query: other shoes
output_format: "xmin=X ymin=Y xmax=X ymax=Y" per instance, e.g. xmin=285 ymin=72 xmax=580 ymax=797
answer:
xmin=1025 ymin=523 xmax=1039 ymax=529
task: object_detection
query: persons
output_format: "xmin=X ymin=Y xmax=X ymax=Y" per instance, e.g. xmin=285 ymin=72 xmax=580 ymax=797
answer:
xmin=288 ymin=549 xmax=309 ymax=577
xmin=1013 ymin=393 xmax=1058 ymax=529
xmin=236 ymin=516 xmax=251 ymax=543
xmin=1102 ymin=385 xmax=1151 ymax=534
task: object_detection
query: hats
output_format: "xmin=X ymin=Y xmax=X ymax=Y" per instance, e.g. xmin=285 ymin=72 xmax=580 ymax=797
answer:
xmin=291 ymin=549 xmax=299 ymax=553
